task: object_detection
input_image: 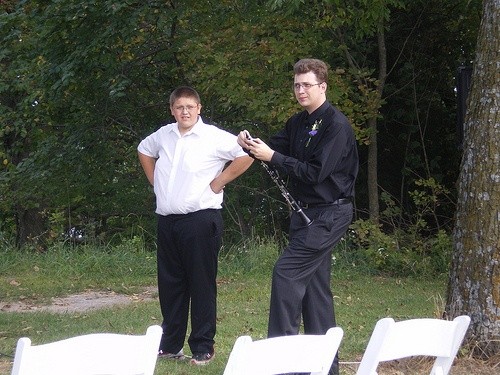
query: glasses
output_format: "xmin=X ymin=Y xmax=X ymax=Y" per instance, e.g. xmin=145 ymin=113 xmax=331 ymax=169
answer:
xmin=172 ymin=104 xmax=199 ymax=111
xmin=295 ymin=82 xmax=321 ymax=90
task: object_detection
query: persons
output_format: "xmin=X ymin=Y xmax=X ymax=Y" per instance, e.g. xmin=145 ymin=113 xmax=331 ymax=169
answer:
xmin=137 ymin=87 xmax=255 ymax=366
xmin=237 ymin=59 xmax=357 ymax=375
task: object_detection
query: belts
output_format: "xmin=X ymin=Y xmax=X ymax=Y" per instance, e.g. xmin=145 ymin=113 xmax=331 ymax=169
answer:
xmin=297 ymin=198 xmax=352 ymax=209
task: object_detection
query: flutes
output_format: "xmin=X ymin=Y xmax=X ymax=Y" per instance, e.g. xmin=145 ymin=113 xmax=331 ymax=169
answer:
xmin=247 ymin=137 xmax=314 ymax=227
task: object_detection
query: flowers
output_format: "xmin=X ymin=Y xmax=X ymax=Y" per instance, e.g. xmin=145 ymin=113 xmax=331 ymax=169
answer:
xmin=309 ymin=130 xmax=317 ymax=136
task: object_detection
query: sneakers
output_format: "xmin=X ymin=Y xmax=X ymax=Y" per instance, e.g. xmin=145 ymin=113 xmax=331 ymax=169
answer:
xmin=158 ymin=348 xmax=186 ymax=360
xmin=189 ymin=347 xmax=215 ymax=364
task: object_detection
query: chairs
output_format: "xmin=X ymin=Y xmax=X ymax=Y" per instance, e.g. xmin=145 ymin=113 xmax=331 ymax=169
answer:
xmin=12 ymin=324 xmax=163 ymax=375
xmin=356 ymin=315 xmax=472 ymax=375
xmin=223 ymin=327 xmax=344 ymax=375
